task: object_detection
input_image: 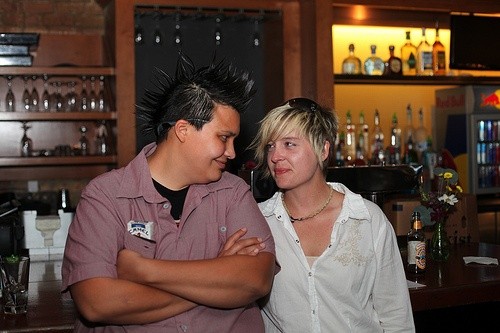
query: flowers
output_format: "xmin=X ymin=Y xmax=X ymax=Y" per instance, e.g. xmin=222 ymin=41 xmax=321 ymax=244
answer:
xmin=419 ymin=167 xmax=463 ymax=224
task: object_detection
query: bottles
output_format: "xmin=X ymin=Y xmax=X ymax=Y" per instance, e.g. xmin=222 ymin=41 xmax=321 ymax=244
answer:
xmin=355 ymin=112 xmax=369 ymax=164
xmin=335 ymin=128 xmax=345 ymax=166
xmin=344 ymin=113 xmax=356 ymax=165
xmin=403 ymin=108 xmax=417 ymax=164
xmin=415 ymin=109 xmax=431 ymax=163
xmin=401 ymin=31 xmax=417 ymax=75
xmin=406 ymin=212 xmax=426 ymax=275
xmin=384 ymin=46 xmax=402 ymax=74
xmin=418 ymin=27 xmax=433 ymax=76
xmin=431 ymin=21 xmax=446 ymax=76
xmin=363 ymin=45 xmax=384 ymax=76
xmin=371 ymin=113 xmax=384 ymax=164
xmin=387 ymin=117 xmax=401 ymax=163
xmin=341 ymin=44 xmax=361 ymax=75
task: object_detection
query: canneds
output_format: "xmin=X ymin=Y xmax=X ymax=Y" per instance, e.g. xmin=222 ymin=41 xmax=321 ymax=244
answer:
xmin=477 ymin=120 xmax=500 ymax=187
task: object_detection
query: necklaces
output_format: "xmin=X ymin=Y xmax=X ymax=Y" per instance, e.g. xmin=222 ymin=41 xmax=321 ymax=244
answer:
xmin=282 ymin=187 xmax=335 ymax=224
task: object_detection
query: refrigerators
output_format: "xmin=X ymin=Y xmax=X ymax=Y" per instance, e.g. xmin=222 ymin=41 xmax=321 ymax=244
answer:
xmin=435 ymin=86 xmax=500 ymax=238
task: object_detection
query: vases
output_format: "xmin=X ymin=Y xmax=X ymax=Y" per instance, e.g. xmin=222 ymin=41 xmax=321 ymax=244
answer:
xmin=430 ymin=221 xmax=450 ymax=287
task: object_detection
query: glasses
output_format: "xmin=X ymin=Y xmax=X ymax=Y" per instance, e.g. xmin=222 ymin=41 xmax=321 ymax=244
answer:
xmin=277 ymin=97 xmax=330 ymax=135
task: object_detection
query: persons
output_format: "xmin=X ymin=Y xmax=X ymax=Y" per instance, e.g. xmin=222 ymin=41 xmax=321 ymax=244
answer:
xmin=60 ymin=78 xmax=280 ymax=332
xmin=246 ymin=98 xmax=416 ymax=333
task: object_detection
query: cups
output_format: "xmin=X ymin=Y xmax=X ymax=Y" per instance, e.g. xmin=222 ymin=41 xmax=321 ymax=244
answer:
xmin=0 ymin=255 xmax=30 ymax=313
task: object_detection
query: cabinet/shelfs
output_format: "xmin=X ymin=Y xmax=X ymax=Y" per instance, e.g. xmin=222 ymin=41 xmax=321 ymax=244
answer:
xmin=0 ymin=66 xmax=117 ymax=165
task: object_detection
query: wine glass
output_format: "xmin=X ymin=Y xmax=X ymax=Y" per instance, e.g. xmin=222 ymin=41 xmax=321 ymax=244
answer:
xmin=19 ymin=121 xmax=32 ymax=156
xmin=95 ymin=120 xmax=109 ymax=155
xmin=3 ymin=75 xmax=109 ymax=113
xmin=77 ymin=127 xmax=91 ymax=155
